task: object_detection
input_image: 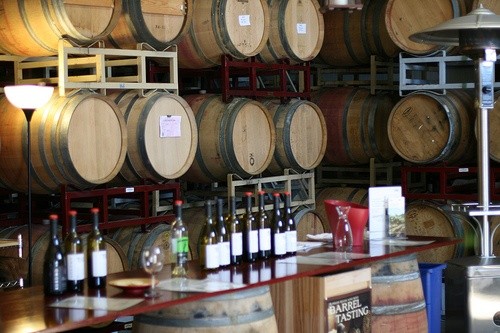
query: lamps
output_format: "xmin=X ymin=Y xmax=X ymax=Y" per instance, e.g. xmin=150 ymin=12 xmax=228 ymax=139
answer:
xmin=409 ymin=3 xmax=500 ymax=61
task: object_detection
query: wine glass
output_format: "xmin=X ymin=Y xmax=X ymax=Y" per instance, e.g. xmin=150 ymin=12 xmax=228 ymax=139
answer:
xmin=141 ymin=246 xmax=166 ymax=299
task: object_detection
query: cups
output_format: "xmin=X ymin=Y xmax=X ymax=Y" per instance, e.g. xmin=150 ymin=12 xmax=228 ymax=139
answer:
xmin=323 ymin=199 xmax=369 ymax=245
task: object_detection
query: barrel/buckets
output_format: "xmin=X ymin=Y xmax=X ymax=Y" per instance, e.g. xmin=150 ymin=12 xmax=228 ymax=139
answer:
xmin=239 ymin=0 xmax=325 ymax=65
xmin=69 ymin=0 xmax=193 ymax=60
xmin=0 ymin=0 xmax=122 ymax=58
xmin=1 ymin=86 xmax=127 ymax=196
xmin=179 ymin=94 xmax=276 ymax=185
xmin=307 ymin=0 xmax=500 ymax=70
xmin=94 ymin=89 xmax=198 ymax=188
xmin=261 ymin=86 xmax=500 ymax=176
xmin=152 ymin=0 xmax=271 ymax=69
xmin=1 ymin=187 xmax=500 ymax=333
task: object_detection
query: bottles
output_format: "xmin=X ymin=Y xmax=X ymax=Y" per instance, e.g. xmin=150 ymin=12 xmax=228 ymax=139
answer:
xmin=255 ymin=191 xmax=271 ymax=260
xmin=270 ymin=193 xmax=288 ymax=257
xmin=334 ymin=206 xmax=354 ymax=252
xmin=243 ymin=191 xmax=259 ymax=261
xmin=65 ymin=210 xmax=83 ymax=294
xmin=225 ymin=196 xmax=243 ymax=265
xmin=283 ymin=192 xmax=297 ymax=255
xmin=197 ymin=200 xmax=220 ymax=270
xmin=169 ymin=200 xmax=190 ymax=277
xmin=213 ymin=199 xmax=231 ymax=267
xmin=43 ymin=214 xmax=64 ymax=297
xmin=87 ymin=208 xmax=107 ymax=288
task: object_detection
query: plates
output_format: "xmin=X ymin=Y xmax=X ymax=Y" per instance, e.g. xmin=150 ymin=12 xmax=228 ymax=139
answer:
xmin=109 ymin=278 xmax=159 ymax=294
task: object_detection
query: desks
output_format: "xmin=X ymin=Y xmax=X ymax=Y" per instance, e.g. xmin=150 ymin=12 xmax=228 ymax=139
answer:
xmin=0 ymin=221 xmax=464 ymax=333
